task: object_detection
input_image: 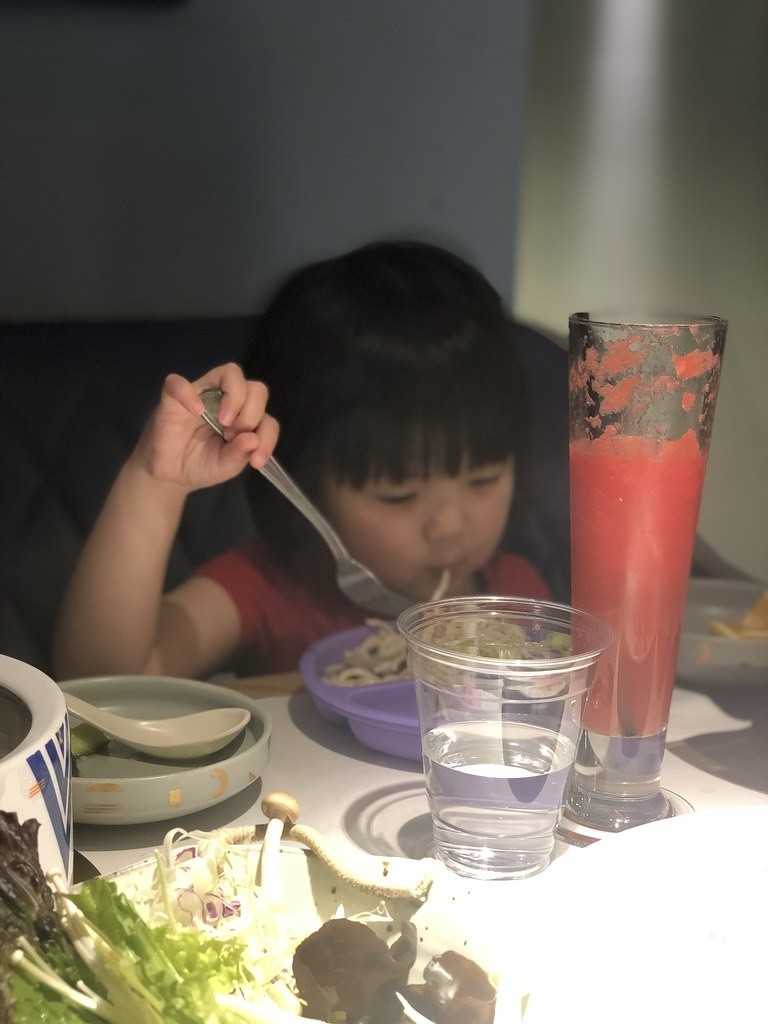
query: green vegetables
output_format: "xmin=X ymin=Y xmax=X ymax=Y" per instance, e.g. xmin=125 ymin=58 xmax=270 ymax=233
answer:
xmin=0 ymin=812 xmax=269 ymax=1024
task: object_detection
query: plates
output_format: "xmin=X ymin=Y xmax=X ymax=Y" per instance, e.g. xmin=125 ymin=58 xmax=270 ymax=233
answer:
xmin=56 ymin=674 xmax=273 ymax=825
xmin=299 ymin=618 xmax=424 ymax=763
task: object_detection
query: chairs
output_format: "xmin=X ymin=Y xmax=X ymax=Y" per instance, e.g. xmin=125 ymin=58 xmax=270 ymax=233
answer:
xmin=0 ymin=310 xmax=753 ymax=682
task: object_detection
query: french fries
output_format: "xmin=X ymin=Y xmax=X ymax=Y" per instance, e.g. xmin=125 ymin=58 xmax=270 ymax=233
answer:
xmin=709 ymin=589 xmax=768 ymax=640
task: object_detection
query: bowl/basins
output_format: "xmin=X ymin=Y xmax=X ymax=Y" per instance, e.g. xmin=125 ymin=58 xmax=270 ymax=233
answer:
xmin=673 ymin=575 xmax=767 ymax=691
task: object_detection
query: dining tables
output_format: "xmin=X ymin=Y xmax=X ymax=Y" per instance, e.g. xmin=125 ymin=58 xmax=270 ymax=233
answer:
xmin=70 ymin=671 xmax=768 ymax=1024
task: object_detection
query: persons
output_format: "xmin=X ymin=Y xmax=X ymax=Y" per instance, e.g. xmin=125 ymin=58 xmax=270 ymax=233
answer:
xmin=44 ymin=236 xmax=554 ymax=699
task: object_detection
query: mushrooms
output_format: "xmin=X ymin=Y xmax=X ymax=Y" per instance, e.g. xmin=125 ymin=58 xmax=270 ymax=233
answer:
xmin=258 ymin=790 xmax=300 ymax=886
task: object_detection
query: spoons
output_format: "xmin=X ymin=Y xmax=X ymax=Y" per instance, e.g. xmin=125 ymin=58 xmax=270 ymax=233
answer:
xmin=64 ymin=690 xmax=251 ymax=759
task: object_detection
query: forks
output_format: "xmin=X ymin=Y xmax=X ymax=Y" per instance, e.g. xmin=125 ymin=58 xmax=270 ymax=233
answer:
xmin=197 ymin=387 xmax=416 ymax=616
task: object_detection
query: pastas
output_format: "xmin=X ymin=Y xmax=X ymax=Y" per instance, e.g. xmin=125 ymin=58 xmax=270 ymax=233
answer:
xmin=320 ymin=568 xmax=536 ymax=688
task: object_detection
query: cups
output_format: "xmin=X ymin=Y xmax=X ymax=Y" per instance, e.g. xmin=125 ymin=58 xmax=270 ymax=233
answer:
xmin=0 ymin=652 xmax=75 ymax=890
xmin=396 ymin=594 xmax=616 ymax=881
xmin=555 ymin=307 xmax=729 ymax=842
